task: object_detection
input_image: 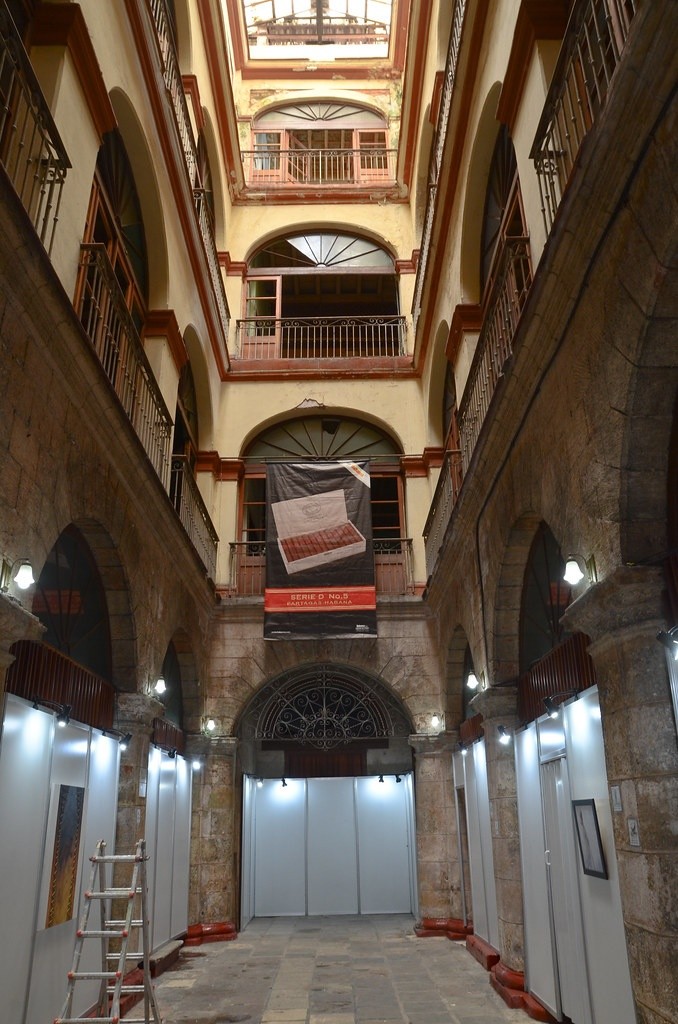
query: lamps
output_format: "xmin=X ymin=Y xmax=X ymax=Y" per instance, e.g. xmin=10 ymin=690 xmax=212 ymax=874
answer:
xmin=257 ymin=777 xmax=263 ymax=787
xmin=101 ymin=727 xmax=133 ymax=750
xmin=147 ymin=673 xmax=167 ymax=694
xmin=458 ymin=741 xmax=468 ymax=755
xmin=202 ymin=715 xmax=215 ymax=730
xmin=168 ymin=746 xmax=177 ymax=758
xmin=467 ymin=668 xmax=485 ymax=690
xmin=497 ymin=719 xmax=529 ymax=742
xmin=0 ymin=557 xmax=35 ymax=592
xmin=395 ymin=775 xmax=402 ymax=783
xmin=431 ymin=712 xmax=444 ymax=729
xmin=541 ymin=689 xmax=579 ymax=719
xmin=562 ymin=552 xmax=597 ymax=585
xmin=378 ymin=775 xmax=384 ymax=783
xmin=32 ymin=696 xmax=72 ymax=727
xmin=657 ymin=622 xmax=678 ymax=660
xmin=280 ymin=778 xmax=287 ymax=787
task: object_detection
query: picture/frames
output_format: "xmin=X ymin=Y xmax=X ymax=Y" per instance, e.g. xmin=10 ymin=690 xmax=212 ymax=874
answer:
xmin=571 ymin=798 xmax=608 ymax=880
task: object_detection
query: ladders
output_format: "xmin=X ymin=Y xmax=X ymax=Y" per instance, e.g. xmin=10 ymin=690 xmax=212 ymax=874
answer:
xmin=54 ymin=837 xmax=163 ymax=1024
